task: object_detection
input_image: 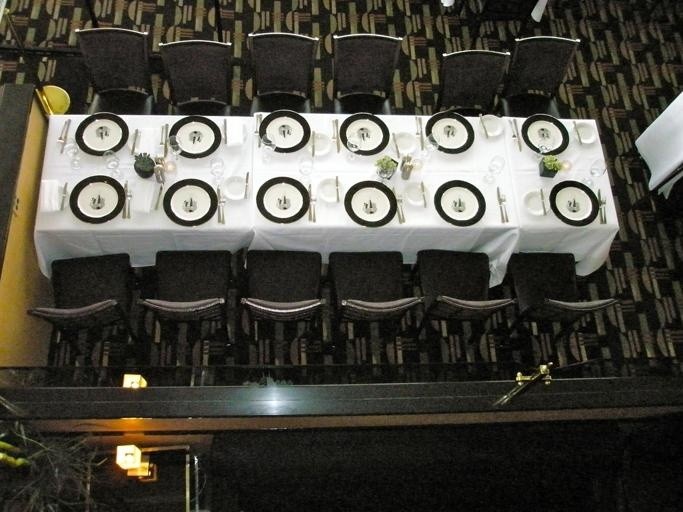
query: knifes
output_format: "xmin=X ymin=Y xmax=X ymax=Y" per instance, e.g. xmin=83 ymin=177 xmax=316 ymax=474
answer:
xmin=393 ymin=133 xmax=402 ymax=159
xmin=496 ymin=186 xmax=506 ymax=223
xmin=335 ymin=175 xmax=340 ymax=203
xmin=164 ymin=124 xmax=170 ymax=159
xmin=310 ymin=130 xmax=315 ymax=158
xmin=391 ymin=186 xmax=402 ymax=223
xmin=121 ymin=180 xmax=128 ymax=219
xmin=420 ymin=180 xmax=428 ymax=209
xmin=217 ymin=184 xmax=222 ymax=223
xmin=307 ymin=182 xmax=314 ymax=222
xmin=334 ymin=119 xmax=340 ymax=153
xmin=597 ymin=188 xmax=604 ymax=225
xmin=130 ymin=129 xmax=139 ymax=155
xmin=513 ymin=118 xmax=523 ymax=152
xmin=60 ymin=119 xmax=72 ymax=155
xmin=479 ymin=112 xmax=490 ymax=139
xmin=244 ymin=171 xmax=250 ymax=199
xmin=59 ymin=182 xmax=68 ymax=212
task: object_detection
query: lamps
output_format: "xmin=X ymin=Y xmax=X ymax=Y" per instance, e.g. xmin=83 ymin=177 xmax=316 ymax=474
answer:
xmin=113 ymin=444 xmax=157 ymax=484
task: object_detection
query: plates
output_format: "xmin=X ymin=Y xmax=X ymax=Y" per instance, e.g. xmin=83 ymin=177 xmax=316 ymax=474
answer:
xmin=162 ymin=179 xmax=218 ymax=226
xmin=339 ymin=113 xmax=390 ymax=156
xmin=434 ymin=180 xmax=487 ymax=226
xmin=256 ymin=176 xmax=311 ymax=224
xmin=425 ymin=112 xmax=475 ymax=153
xmin=344 ymin=180 xmax=397 ymax=228
xmin=258 ymin=110 xmax=310 ymax=153
xmin=307 ymin=131 xmax=332 ymax=156
xmin=521 ymin=113 xmax=569 ymax=156
xmin=392 ymin=132 xmax=418 ymax=154
xmin=573 ymin=122 xmax=597 ymax=145
xmin=68 ymin=175 xmax=126 ymax=224
xmin=523 ymin=191 xmax=548 ymax=217
xmin=549 ymin=180 xmax=600 ymax=226
xmin=478 ymin=114 xmax=505 ymax=137
xmin=318 ymin=178 xmax=343 ymax=205
xmin=403 ymin=182 xmax=430 ymax=207
xmin=168 ymin=115 xmax=221 ymax=159
xmin=75 ymin=111 xmax=128 ymax=156
xmin=221 ymin=176 xmax=250 ymax=200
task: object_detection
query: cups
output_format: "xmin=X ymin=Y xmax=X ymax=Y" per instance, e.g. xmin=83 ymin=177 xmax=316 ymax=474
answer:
xmin=259 ymin=133 xmax=277 ymax=154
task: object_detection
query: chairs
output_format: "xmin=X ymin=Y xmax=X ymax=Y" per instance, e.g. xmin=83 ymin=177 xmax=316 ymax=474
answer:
xmin=157 ymin=39 xmax=235 ymax=115
xmin=412 ymin=251 xmax=518 ymax=345
xmin=326 ymin=252 xmax=424 ymax=345
xmin=241 ymin=251 xmax=326 ymax=346
xmin=499 ymin=251 xmax=613 ymax=345
xmin=511 ymin=35 xmax=581 ymax=114
xmin=248 ymin=31 xmax=319 ymax=112
xmin=71 ymin=27 xmax=155 ymax=115
xmin=331 ymin=34 xmax=403 ymax=114
xmin=138 ymin=251 xmax=236 ymax=349
xmin=435 ymin=50 xmax=511 ymax=113
xmin=26 ymin=250 xmax=146 ymax=353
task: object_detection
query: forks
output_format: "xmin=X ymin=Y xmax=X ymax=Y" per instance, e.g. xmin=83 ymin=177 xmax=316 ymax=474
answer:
xmin=398 ymin=194 xmax=406 ymax=223
xmin=125 ymin=189 xmax=133 ymax=219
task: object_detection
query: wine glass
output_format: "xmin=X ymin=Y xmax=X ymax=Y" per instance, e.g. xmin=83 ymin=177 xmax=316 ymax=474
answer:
xmin=101 ymin=150 xmax=122 ymax=178
xmin=582 ymin=159 xmax=608 ymax=187
